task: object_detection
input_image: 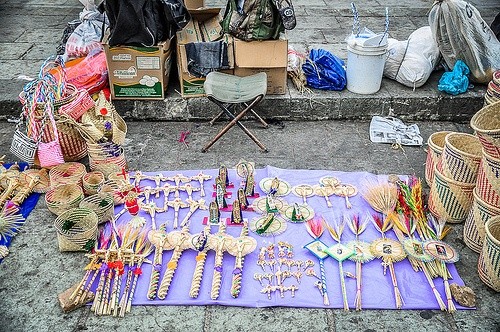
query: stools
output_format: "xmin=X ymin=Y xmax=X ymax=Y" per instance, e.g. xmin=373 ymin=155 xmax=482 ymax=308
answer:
xmin=198 ymin=70 xmax=273 ymax=155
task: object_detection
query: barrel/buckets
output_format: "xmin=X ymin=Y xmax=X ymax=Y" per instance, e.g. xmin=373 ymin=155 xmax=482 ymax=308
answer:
xmin=345 ymin=33 xmax=389 ymax=95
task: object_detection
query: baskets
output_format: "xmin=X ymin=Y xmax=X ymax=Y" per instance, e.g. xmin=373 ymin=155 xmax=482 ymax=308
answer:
xmin=424 ymin=69 xmax=500 ymax=294
xmin=18 ymin=83 xmax=131 ymax=252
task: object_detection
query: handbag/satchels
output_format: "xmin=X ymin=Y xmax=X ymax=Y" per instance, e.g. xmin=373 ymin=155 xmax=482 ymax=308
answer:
xmin=10 ymin=80 xmax=65 ymax=168
xmin=219 ymin=0 xmax=297 ymax=42
xmin=97 ymin=0 xmax=191 ymax=50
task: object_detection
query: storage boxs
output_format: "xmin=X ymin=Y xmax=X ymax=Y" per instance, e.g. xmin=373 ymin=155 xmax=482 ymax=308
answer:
xmin=177 ymin=7 xmax=235 ymax=97
xmin=232 ymin=35 xmax=288 ymax=95
xmin=96 ymin=34 xmax=175 ymax=101
